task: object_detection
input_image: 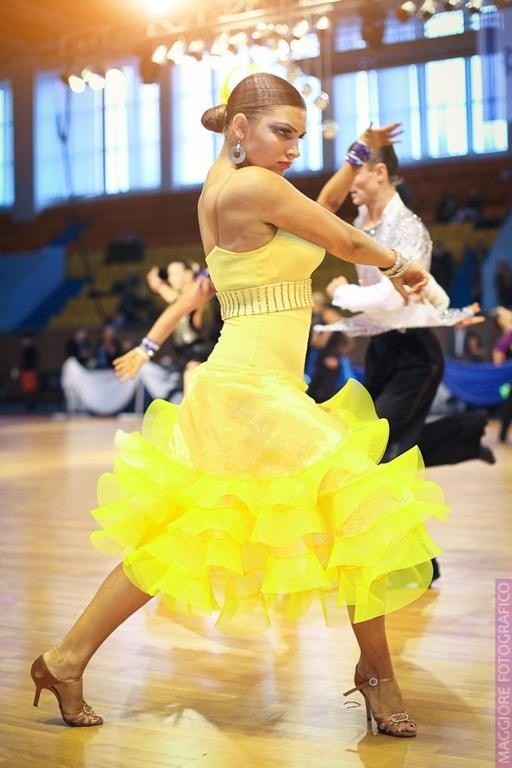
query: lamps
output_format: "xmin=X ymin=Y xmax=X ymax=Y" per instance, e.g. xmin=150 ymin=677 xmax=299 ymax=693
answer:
xmin=58 ymin=0 xmax=512 ymax=94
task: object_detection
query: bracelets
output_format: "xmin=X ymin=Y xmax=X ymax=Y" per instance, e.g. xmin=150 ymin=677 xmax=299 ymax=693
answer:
xmin=347 ymin=140 xmax=372 ymax=167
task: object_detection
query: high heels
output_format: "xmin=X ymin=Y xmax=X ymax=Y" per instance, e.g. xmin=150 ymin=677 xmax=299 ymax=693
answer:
xmin=30 ymin=655 xmax=104 ymax=726
xmin=345 ymin=663 xmax=419 ymax=738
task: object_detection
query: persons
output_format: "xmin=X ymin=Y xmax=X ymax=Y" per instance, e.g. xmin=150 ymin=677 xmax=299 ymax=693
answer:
xmin=32 ymin=74 xmax=431 ymax=738
xmin=8 ymin=189 xmax=512 ymax=441
xmin=311 ymin=146 xmax=497 ymax=585
